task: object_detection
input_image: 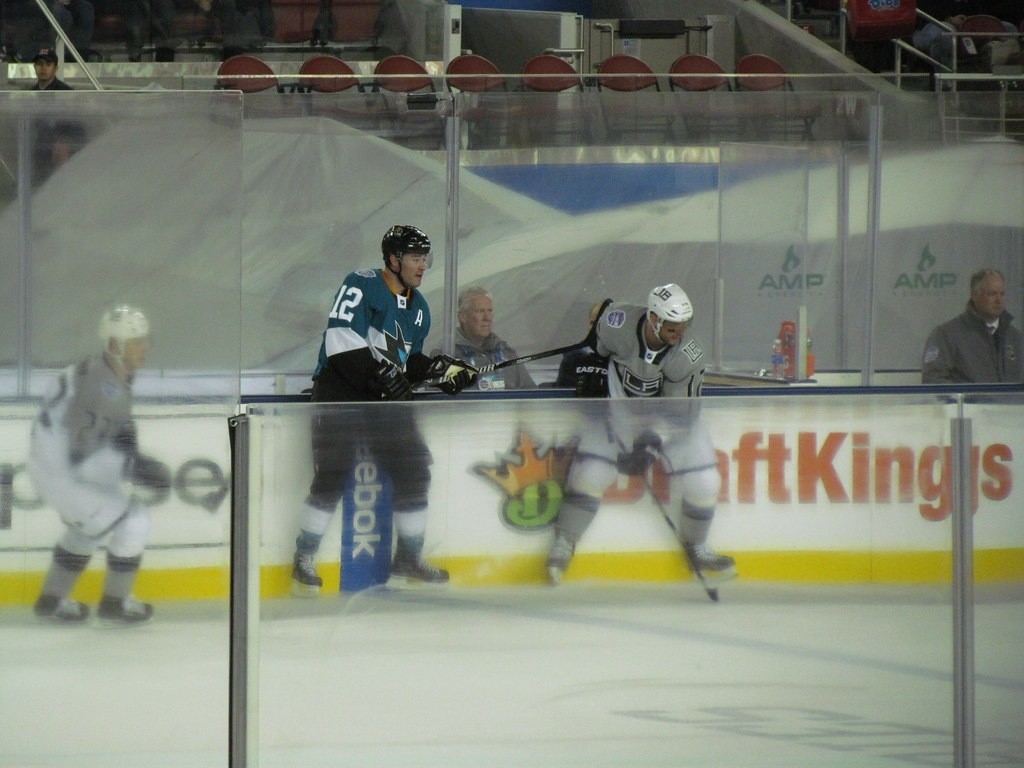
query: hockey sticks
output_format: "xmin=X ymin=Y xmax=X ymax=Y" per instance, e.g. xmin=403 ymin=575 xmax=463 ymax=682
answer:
xmin=381 ymin=299 xmax=614 ymax=399
xmin=145 ymin=472 xmax=231 ymax=489
xmin=604 ymin=416 xmax=719 ymax=603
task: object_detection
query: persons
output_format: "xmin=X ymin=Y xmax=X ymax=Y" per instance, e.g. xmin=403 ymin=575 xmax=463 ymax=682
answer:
xmin=555 ymin=305 xmax=600 ymax=385
xmin=290 ymin=225 xmax=479 ymax=600
xmin=27 ymin=308 xmax=171 ymax=627
xmin=548 ymin=284 xmax=739 ymax=586
xmin=31 ymin=50 xmax=84 ymax=183
xmin=428 ymin=287 xmax=538 ymax=389
xmin=218 ymin=0 xmax=275 ymax=60
xmin=24 ymin=0 xmax=95 ymax=61
xmin=116 ymin=0 xmax=176 ymax=62
xmin=922 ymin=269 xmax=1024 ymax=401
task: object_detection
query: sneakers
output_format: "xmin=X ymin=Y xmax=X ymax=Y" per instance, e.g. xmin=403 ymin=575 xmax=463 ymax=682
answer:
xmin=289 ymin=552 xmax=323 ymax=598
xmin=386 ymin=548 xmax=450 ymax=592
xmin=91 ymin=595 xmax=157 ymax=629
xmin=681 ymin=540 xmax=737 ymax=579
xmin=545 ymin=537 xmax=575 ymax=584
xmin=27 ymin=595 xmax=92 ymax=627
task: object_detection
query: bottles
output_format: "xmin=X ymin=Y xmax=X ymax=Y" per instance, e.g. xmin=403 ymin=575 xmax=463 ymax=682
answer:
xmin=770 ymin=339 xmax=786 ymax=378
xmin=806 ymin=338 xmax=815 ymax=377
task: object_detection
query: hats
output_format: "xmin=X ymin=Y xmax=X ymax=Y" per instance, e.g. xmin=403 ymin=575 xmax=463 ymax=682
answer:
xmin=32 ymin=48 xmax=58 ymax=63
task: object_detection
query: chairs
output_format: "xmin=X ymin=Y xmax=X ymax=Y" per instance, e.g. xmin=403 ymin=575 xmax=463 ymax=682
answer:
xmin=959 ymin=15 xmax=1024 ymax=133
xmin=0 ymin=0 xmax=822 ymax=149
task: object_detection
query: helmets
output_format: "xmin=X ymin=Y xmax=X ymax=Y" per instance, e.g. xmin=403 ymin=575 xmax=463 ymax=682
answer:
xmin=381 ymin=225 xmax=431 ymax=260
xmin=96 ymin=307 xmax=152 ymax=341
xmin=647 ymin=283 xmax=693 ymax=323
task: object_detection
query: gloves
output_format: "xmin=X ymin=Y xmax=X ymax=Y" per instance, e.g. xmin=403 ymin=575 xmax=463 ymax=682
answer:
xmin=375 ymin=363 xmax=412 ymax=400
xmin=422 ymin=354 xmax=479 ymax=395
